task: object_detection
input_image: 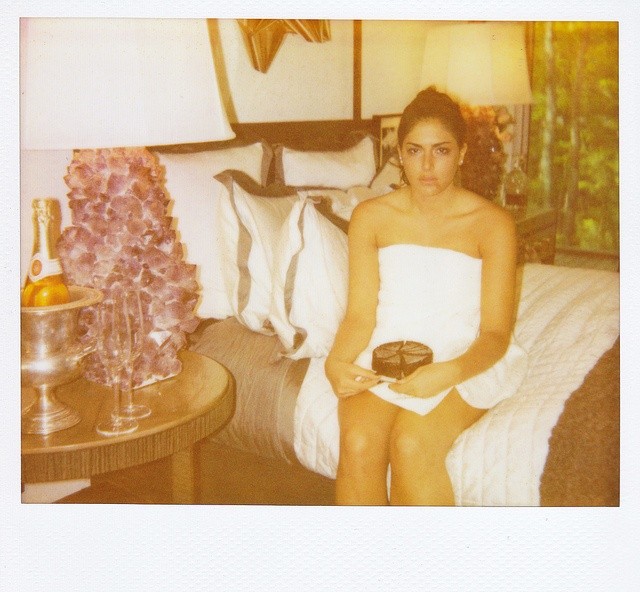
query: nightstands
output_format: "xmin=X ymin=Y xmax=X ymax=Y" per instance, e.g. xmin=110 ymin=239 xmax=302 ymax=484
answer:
xmin=506 ymin=207 xmax=559 ymax=264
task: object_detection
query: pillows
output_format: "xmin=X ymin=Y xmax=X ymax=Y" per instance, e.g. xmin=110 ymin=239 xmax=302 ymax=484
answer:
xmin=155 ymin=139 xmax=273 ymax=320
xmin=270 ymin=195 xmax=348 ymax=362
xmin=279 ymin=136 xmax=378 ymax=190
xmin=210 ymin=170 xmax=345 ymax=336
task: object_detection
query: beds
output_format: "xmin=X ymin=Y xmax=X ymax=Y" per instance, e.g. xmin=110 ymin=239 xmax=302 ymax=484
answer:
xmin=144 ymin=117 xmax=620 ymax=507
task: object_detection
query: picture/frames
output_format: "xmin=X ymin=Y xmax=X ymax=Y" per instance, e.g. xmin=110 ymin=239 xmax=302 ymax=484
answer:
xmin=373 ymin=114 xmax=403 ymax=172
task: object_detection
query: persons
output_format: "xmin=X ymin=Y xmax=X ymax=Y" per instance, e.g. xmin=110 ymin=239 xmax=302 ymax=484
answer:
xmin=322 ymin=86 xmax=519 ymax=504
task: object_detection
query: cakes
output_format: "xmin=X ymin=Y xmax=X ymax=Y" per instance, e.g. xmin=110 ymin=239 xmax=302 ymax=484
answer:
xmin=372 ymin=341 xmax=433 ymax=379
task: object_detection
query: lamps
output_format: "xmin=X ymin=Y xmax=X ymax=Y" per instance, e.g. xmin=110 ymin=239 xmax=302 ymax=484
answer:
xmin=421 ymin=21 xmax=539 ymax=203
xmin=20 ymin=17 xmax=238 ymax=388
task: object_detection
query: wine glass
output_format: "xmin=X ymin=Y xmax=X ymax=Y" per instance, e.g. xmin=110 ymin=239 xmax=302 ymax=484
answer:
xmin=93 ymin=296 xmax=139 ymax=441
xmin=110 ymin=297 xmax=154 ymax=424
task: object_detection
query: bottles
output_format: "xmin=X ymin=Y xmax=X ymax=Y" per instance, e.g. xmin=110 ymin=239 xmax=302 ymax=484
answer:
xmin=502 ymin=153 xmax=529 ymax=210
xmin=24 ymin=197 xmax=69 ymax=288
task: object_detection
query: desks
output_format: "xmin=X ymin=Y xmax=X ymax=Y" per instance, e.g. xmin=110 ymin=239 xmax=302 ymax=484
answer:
xmin=22 ymin=352 xmax=237 ymax=504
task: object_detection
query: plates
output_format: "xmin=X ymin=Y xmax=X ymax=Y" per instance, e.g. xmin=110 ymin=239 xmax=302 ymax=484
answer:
xmin=372 ymin=369 xmax=397 ymax=384
